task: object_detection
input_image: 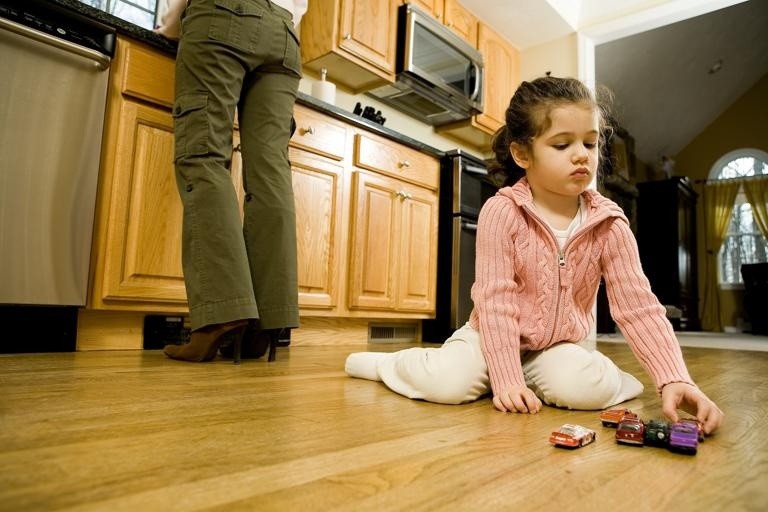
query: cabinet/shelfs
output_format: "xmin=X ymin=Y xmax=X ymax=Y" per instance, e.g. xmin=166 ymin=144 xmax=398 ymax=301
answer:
xmin=637 ymin=177 xmax=701 ymax=330
xmin=299 ymin=0 xmax=398 ymax=77
xmin=343 ymin=132 xmax=441 ymax=319
xmin=99 ymin=44 xmax=347 ymax=313
xmin=405 ymin=0 xmax=479 ymax=50
xmin=435 ymin=22 xmax=519 ymax=133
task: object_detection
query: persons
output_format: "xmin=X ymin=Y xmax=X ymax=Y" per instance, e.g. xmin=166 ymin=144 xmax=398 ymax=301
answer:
xmin=345 ymin=70 xmax=725 ymax=436
xmin=152 ymin=0 xmax=309 ymax=364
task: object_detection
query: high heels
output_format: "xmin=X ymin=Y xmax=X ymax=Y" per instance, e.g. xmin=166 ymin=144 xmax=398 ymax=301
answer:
xmin=219 ymin=329 xmax=284 ymax=361
xmin=163 ymin=319 xmax=249 ymax=365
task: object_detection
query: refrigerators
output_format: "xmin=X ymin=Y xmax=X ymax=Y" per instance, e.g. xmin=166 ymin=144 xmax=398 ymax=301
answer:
xmin=420 ymin=150 xmax=501 ymax=346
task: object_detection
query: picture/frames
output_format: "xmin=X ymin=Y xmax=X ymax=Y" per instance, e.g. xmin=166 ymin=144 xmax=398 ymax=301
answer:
xmin=604 ymin=115 xmax=637 ymax=185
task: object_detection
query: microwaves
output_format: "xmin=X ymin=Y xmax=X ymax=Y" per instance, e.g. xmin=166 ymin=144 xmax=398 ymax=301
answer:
xmin=361 ymin=3 xmax=487 ymax=129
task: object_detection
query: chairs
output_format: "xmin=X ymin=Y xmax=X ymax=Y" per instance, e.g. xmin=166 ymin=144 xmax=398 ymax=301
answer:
xmin=737 ymin=261 xmax=768 ymax=335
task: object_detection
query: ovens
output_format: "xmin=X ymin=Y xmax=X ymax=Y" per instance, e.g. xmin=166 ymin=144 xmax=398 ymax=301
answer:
xmin=0 ymin=0 xmax=117 ymax=307
